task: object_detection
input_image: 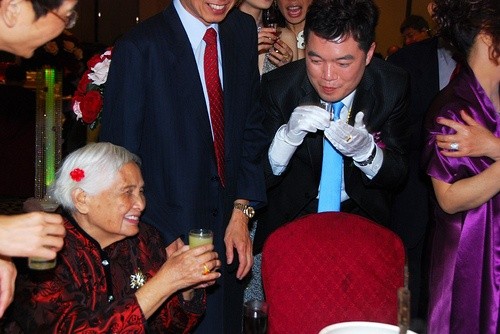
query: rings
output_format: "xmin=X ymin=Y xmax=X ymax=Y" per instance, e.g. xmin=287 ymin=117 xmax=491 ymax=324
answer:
xmin=284 ymin=54 xmax=289 ymax=59
xmin=202 ymin=264 xmax=211 ymax=274
xmin=451 ymin=143 xmax=459 ymax=150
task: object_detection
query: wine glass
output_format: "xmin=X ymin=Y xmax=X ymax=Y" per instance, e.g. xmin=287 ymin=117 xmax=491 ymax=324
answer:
xmin=268 ymin=23 xmax=282 ymax=54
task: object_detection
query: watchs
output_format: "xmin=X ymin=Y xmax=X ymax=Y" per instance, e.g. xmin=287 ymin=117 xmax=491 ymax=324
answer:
xmin=233 ymin=202 xmax=255 ymax=219
xmin=355 ymin=145 xmax=378 ymax=167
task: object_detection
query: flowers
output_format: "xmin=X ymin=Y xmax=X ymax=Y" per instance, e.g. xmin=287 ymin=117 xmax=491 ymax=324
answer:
xmin=71 ymin=45 xmax=112 ymax=132
xmin=70 ymin=169 xmax=85 ymax=181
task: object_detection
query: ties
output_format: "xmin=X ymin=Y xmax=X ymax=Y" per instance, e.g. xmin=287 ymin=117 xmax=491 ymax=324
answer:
xmin=203 ymin=28 xmax=225 ymax=188
xmin=318 ymin=102 xmax=344 ymax=213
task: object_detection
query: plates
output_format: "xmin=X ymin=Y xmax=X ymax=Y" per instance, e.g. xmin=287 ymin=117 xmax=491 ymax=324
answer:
xmin=318 ymin=321 xmax=417 ymax=334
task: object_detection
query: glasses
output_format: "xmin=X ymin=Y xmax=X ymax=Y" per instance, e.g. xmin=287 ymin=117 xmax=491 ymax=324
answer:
xmin=50 ymin=9 xmax=78 ymax=28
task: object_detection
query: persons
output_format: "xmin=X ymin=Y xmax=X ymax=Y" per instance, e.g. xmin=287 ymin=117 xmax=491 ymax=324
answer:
xmin=408 ymin=0 xmax=500 ymax=334
xmin=1 ymin=0 xmax=84 ymax=331
xmin=432 ymin=108 xmax=500 ymax=167
xmin=1 ymin=138 xmax=222 ymax=333
xmin=382 ymin=0 xmax=459 ymax=114
xmin=230 ymin=0 xmax=424 ymax=322
xmin=236 ymin=0 xmax=313 ymax=78
xmin=399 ymin=15 xmax=431 ymax=47
xmin=101 ymin=1 xmax=262 ymax=334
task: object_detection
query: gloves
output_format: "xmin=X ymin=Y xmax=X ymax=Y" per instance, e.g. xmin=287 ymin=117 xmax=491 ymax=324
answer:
xmin=284 ymin=104 xmax=334 ymax=144
xmin=323 ymin=111 xmax=375 ymax=163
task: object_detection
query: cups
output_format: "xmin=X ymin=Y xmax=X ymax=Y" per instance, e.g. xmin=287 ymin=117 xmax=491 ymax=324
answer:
xmin=189 ymin=228 xmax=214 ymax=252
xmin=27 ymin=202 xmax=57 ymax=270
xmin=320 ymin=103 xmax=331 ymax=128
xmin=243 ymin=300 xmax=266 ymax=334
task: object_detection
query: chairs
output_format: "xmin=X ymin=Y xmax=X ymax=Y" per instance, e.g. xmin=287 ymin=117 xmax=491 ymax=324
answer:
xmin=261 ymin=212 xmax=405 ymax=334
